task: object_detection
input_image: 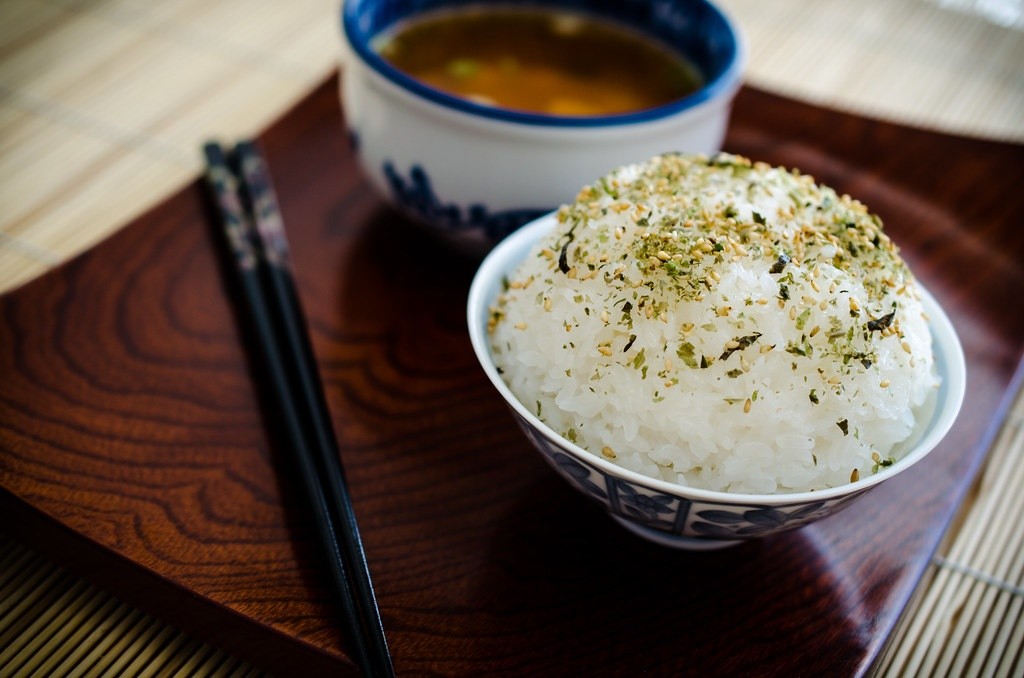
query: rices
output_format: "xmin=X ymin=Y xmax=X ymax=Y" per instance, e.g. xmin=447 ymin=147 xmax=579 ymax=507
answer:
xmin=483 ymin=150 xmax=937 ymax=494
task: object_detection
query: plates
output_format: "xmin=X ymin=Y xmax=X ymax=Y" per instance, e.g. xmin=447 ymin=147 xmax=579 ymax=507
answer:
xmin=0 ymin=62 xmax=1024 ymax=677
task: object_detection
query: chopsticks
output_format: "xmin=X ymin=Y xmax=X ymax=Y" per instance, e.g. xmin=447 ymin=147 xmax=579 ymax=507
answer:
xmin=203 ymin=138 xmax=395 ymax=678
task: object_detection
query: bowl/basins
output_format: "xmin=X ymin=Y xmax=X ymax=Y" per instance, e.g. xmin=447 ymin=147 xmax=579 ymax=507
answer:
xmin=467 ymin=212 xmax=965 ymax=551
xmin=337 ymin=1 xmax=748 ymax=259
